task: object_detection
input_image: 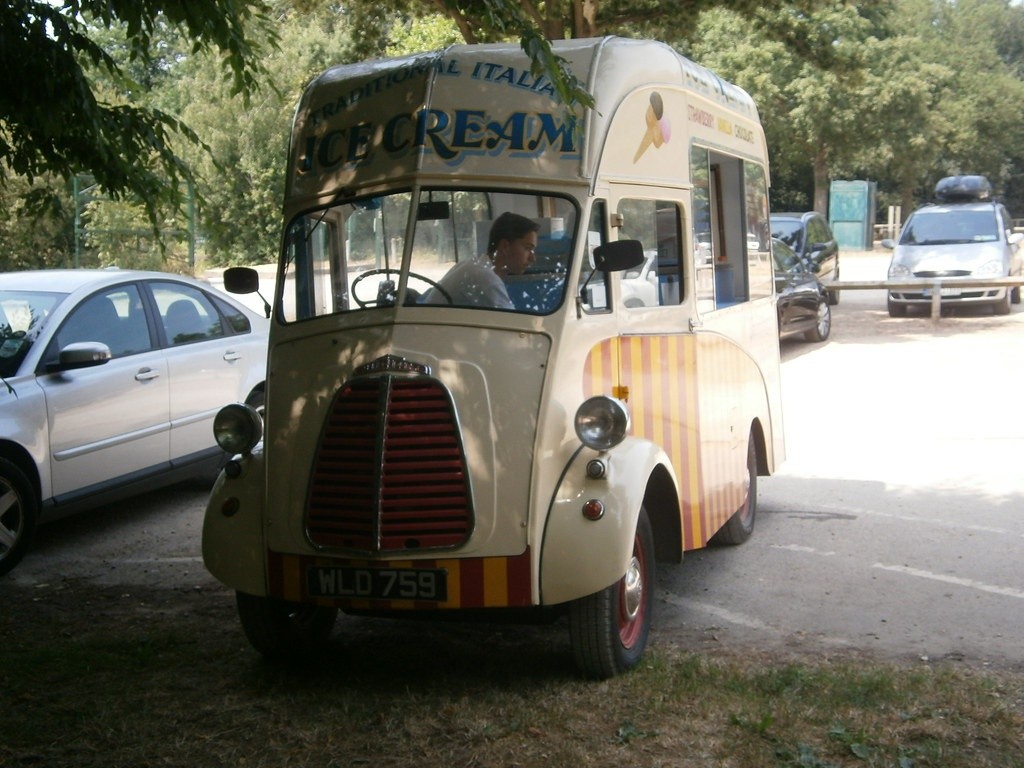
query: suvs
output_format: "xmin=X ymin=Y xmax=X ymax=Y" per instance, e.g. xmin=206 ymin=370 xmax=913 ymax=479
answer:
xmin=880 ymin=174 xmax=1024 ymax=316
xmin=769 ymin=211 xmax=842 ymax=305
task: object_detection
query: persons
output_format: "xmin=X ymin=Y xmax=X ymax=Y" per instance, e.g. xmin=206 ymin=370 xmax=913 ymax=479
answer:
xmin=166 ymin=299 xmax=204 ymax=340
xmin=425 ymin=212 xmax=541 ymax=309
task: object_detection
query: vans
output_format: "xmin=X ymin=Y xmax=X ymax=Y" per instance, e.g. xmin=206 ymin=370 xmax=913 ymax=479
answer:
xmin=202 ymin=27 xmax=787 ymax=683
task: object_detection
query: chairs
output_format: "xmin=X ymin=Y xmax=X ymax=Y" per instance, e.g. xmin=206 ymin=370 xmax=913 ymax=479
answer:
xmin=65 ymin=297 xmax=203 ymax=354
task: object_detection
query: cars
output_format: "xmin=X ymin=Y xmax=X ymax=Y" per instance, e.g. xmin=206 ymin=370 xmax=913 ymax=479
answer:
xmin=694 ymin=231 xmax=833 ymax=343
xmin=0 ymin=268 xmax=271 ymax=576
xmin=579 ymin=247 xmax=661 ymax=309
